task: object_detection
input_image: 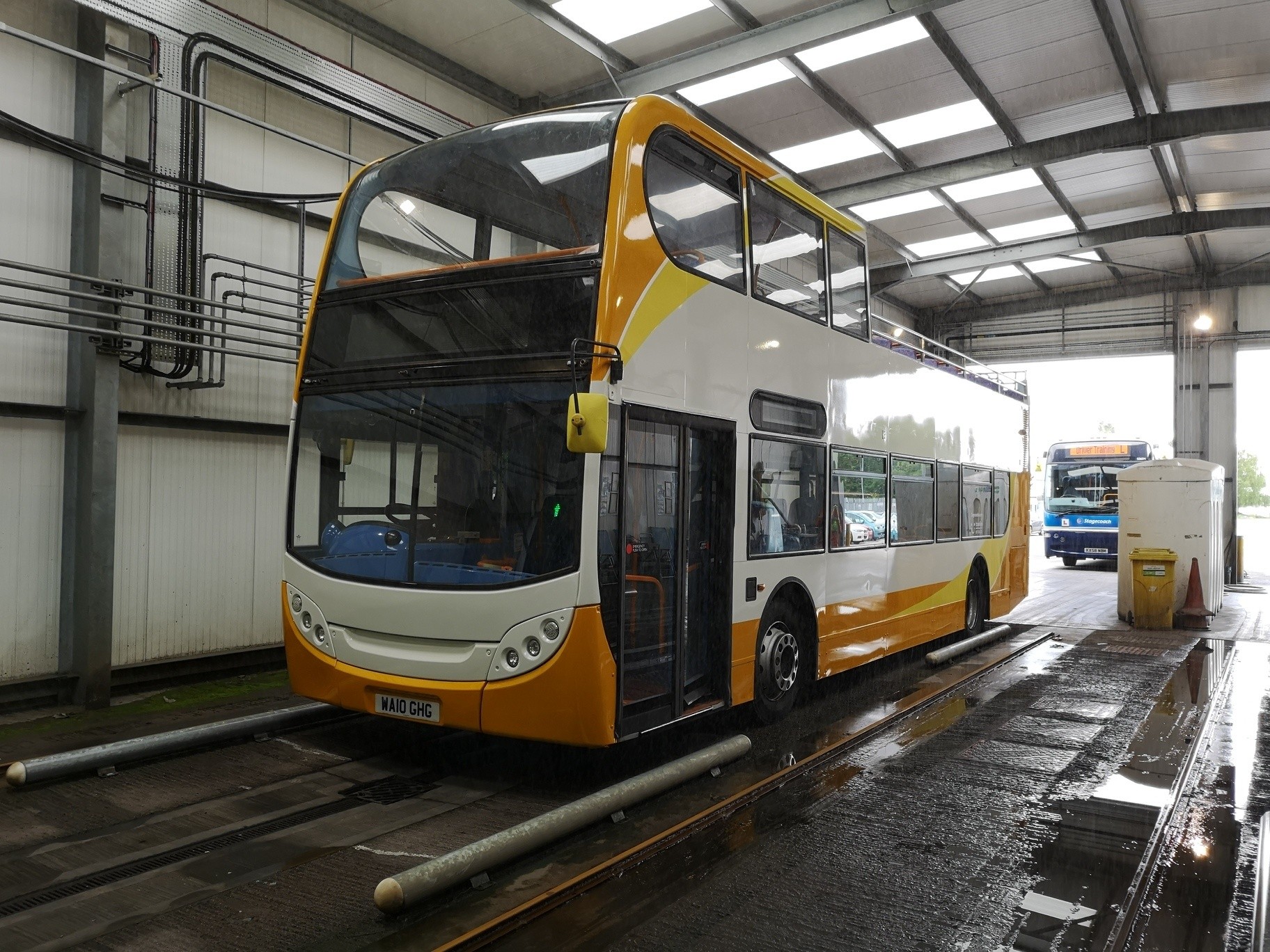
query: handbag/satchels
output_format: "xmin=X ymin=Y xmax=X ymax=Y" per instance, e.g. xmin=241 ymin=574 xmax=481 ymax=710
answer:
xmin=832 ymin=519 xmax=838 ymax=532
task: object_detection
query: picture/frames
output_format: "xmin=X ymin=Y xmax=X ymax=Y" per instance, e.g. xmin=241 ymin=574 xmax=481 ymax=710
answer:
xmin=608 ymin=472 xmax=620 ymax=514
xmin=665 ymin=481 xmax=673 ymax=514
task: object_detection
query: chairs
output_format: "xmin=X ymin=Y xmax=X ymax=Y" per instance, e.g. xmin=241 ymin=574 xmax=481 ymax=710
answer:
xmin=872 ymin=338 xmax=1024 ymax=402
xmin=671 ymin=250 xmax=866 ymax=337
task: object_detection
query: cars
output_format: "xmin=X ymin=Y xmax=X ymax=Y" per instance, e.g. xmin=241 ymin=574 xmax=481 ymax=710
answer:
xmin=1029 ymin=518 xmax=1044 ymax=536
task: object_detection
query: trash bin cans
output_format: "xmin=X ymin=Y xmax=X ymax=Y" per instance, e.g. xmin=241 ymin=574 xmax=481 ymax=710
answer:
xmin=1127 ymin=548 xmax=1179 ymax=631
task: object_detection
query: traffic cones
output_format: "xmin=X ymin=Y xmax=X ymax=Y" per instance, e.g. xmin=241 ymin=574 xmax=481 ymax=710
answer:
xmin=1176 ymin=557 xmax=1215 ymax=617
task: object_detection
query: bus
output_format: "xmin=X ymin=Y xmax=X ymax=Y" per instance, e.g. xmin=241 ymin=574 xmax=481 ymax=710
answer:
xmin=1042 ymin=440 xmax=1156 ymax=568
xmin=278 ymin=93 xmax=1031 ymax=751
